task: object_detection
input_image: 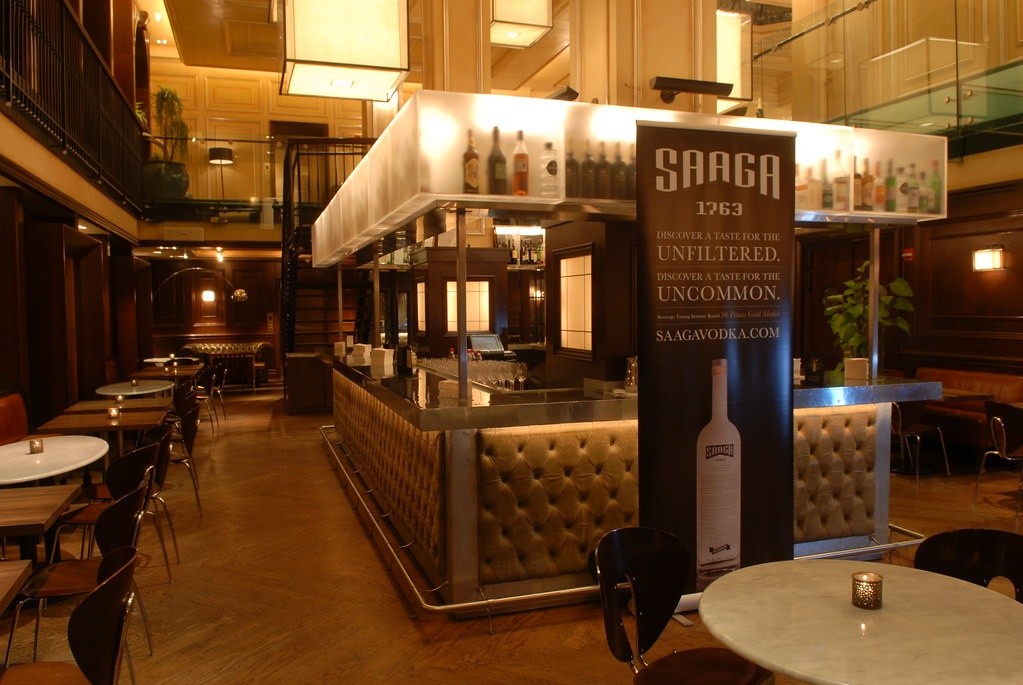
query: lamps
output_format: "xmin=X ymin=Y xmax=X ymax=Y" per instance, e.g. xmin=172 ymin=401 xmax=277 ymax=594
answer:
xmin=279 ymin=1 xmax=409 ymax=102
xmin=208 ymin=147 xmax=234 ymax=201
xmin=972 ymin=245 xmax=1002 ymax=270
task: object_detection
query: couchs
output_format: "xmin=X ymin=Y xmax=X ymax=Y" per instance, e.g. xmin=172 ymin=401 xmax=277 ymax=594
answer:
xmin=915 ymin=367 xmax=1023 ymax=449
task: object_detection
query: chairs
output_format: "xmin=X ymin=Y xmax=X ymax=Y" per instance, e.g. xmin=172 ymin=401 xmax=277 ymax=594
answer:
xmin=0 ymin=358 xmax=231 ymax=685
xmin=914 ymin=529 xmax=1023 ymax=610
xmin=892 ymin=402 xmax=951 ymax=483
xmin=595 ymin=526 xmax=771 ymax=685
xmin=972 ymin=417 xmax=1023 ymax=508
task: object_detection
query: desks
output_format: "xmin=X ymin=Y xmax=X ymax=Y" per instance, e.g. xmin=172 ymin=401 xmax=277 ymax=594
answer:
xmin=144 ymin=365 xmax=204 ymax=374
xmin=0 ymin=436 xmax=109 ymax=489
xmin=129 ymin=371 xmax=199 ymax=385
xmin=63 ymin=397 xmax=173 ymax=418
xmin=0 ymin=484 xmax=81 ymax=570
xmin=95 ymin=380 xmax=174 ymax=400
xmin=697 ymin=559 xmax=1023 ymax=685
xmin=36 ymin=410 xmax=167 ymax=457
xmin=0 ymin=559 xmax=33 ymax=617
xmin=926 ymin=388 xmax=994 ymax=454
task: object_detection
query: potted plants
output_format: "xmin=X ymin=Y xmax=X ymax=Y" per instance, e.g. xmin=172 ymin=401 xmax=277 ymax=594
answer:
xmin=141 ymin=88 xmax=190 ymax=199
xmin=827 ymin=262 xmax=911 ymax=381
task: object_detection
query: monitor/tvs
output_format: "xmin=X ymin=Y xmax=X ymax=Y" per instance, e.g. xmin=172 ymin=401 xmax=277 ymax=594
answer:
xmin=470 ymin=335 xmax=505 ymax=354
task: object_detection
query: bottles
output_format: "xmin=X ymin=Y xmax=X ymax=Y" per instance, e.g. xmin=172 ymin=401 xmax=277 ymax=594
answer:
xmin=488 ymin=126 xmax=507 ymax=195
xmin=513 ymin=130 xmax=529 ymax=195
xmin=499 ymin=239 xmax=544 ymax=265
xmin=464 ymin=130 xmax=479 ymax=194
xmin=754 ymin=98 xmax=764 ymax=118
xmin=539 ymin=143 xmax=559 ymax=198
xmin=467 ymin=349 xmax=471 ymax=360
xmin=696 ymin=359 xmax=741 ymax=592
xmin=795 ymin=150 xmax=942 ymax=215
xmin=449 ymin=348 xmax=455 ymax=359
xmin=470 ymin=350 xmax=475 ymax=360
xmin=566 ymin=139 xmax=636 ymax=200
xmin=475 ymin=351 xmax=482 ymax=361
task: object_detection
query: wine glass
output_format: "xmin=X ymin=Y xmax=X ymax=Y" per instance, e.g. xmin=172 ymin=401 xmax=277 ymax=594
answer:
xmin=415 ymin=356 xmax=529 ymax=391
